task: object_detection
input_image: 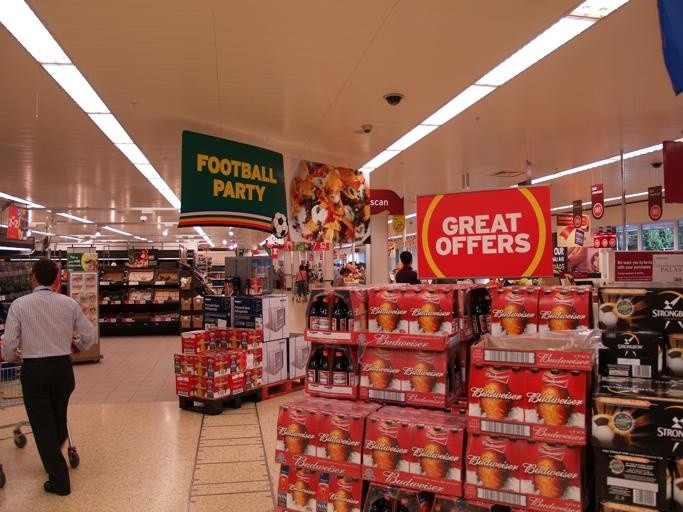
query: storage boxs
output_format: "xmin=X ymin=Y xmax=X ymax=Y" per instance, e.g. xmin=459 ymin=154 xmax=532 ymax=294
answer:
xmin=591 ymin=286 xmax=683 ymax=511
xmin=203 ymin=294 xmax=312 ymax=385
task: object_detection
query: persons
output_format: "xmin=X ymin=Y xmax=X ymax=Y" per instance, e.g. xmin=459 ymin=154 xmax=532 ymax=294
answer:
xmin=271 ymin=250 xmax=457 ymax=305
xmin=592 ymin=251 xmax=599 ymax=271
xmin=0 ymin=258 xmax=96 ymax=497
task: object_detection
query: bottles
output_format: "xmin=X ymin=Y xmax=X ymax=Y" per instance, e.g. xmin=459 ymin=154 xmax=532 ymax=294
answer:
xmin=271 ymin=285 xmax=593 ymax=511
xmin=175 ymin=326 xmax=263 ymax=398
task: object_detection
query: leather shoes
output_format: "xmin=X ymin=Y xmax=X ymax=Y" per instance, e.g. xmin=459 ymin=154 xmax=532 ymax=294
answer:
xmin=44 ymin=480 xmax=70 ymax=495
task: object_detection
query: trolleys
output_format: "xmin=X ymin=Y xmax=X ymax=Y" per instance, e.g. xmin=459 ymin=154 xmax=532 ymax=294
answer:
xmin=0 ymin=338 xmax=80 ymax=487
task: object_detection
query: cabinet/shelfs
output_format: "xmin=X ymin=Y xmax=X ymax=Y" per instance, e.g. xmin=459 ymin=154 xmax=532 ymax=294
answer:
xmin=0 ymin=263 xmax=62 ymax=330
xmin=99 ymin=281 xmax=179 ymax=336
